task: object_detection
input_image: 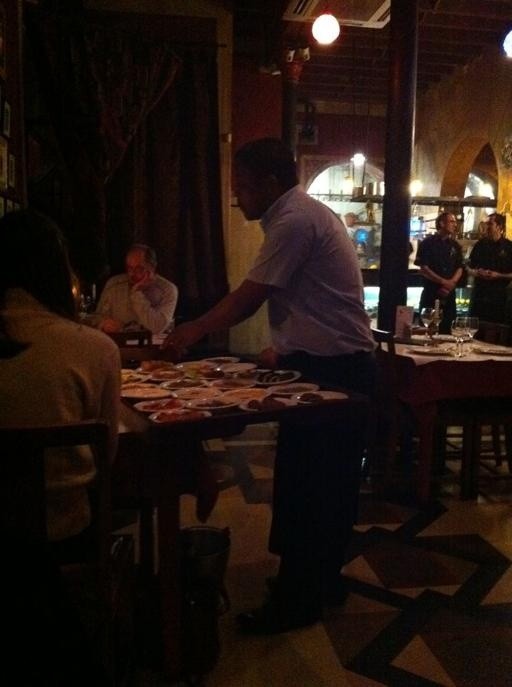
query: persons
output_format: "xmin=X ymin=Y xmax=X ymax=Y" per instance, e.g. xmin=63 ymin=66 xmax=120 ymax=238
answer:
xmin=415 ymin=211 xmax=465 ymax=334
xmin=93 ymin=245 xmax=177 ymax=333
xmin=468 ymin=212 xmax=512 ymax=325
xmin=161 ymin=137 xmax=380 ymax=634
xmin=0 ymin=208 xmax=120 ymax=565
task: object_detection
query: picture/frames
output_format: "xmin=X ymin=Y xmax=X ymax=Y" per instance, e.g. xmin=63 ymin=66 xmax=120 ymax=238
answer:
xmin=0 ymin=2 xmax=21 ymax=219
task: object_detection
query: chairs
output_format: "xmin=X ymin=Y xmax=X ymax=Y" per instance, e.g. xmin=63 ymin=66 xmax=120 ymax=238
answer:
xmin=0 ymin=417 xmax=115 ymax=684
xmin=105 ymin=326 xmax=157 ymax=345
xmin=370 ymin=318 xmax=512 ymax=511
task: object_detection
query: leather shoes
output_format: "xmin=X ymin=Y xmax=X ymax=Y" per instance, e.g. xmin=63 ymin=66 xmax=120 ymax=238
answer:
xmin=239 ymin=593 xmax=323 ymax=634
xmin=263 ymin=574 xmax=349 ymax=607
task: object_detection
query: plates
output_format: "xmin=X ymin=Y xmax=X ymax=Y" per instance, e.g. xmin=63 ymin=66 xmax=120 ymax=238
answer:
xmin=469 ymin=344 xmax=512 ymax=355
xmin=408 ymin=334 xmax=444 ymax=344
xmin=407 ymin=344 xmax=451 ymax=355
xmin=117 ymin=356 xmax=351 ymax=426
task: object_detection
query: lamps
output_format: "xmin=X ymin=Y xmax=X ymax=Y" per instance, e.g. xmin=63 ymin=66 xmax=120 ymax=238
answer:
xmin=346 ymin=27 xmax=377 ymax=189
xmin=309 ymin=0 xmax=344 ymax=47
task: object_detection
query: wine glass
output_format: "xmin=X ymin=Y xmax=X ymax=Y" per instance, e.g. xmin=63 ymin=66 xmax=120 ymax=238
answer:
xmin=75 ymin=281 xmax=92 ymax=325
xmin=419 ymin=306 xmax=479 ymax=358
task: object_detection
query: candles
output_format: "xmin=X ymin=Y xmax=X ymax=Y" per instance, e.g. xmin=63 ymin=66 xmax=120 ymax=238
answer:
xmin=79 ymin=283 xmax=98 ymax=301
